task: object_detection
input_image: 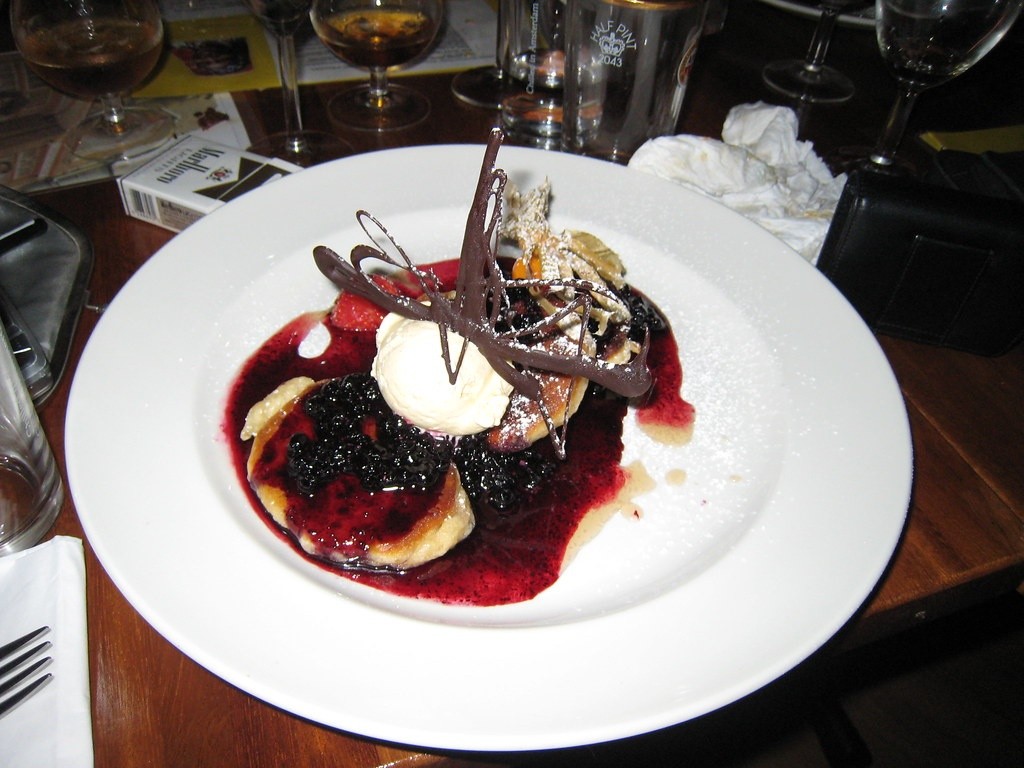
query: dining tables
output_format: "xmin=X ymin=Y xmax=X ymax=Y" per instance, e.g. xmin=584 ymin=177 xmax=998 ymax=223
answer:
xmin=1 ymin=65 xmax=1024 ymax=768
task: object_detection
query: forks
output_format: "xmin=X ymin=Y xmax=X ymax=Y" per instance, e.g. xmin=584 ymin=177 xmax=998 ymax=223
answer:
xmin=0 ymin=626 xmax=52 ymax=720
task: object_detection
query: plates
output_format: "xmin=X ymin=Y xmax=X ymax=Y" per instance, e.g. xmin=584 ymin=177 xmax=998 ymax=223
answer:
xmin=766 ymin=0 xmax=881 ymax=28
xmin=65 ymin=145 xmax=914 ymax=758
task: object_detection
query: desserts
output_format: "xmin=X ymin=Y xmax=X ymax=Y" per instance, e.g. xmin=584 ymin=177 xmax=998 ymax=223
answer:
xmin=234 ymin=121 xmax=656 ymax=572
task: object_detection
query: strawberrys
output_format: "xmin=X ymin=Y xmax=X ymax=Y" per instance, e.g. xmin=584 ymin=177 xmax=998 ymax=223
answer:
xmin=330 ymin=274 xmax=401 ymax=331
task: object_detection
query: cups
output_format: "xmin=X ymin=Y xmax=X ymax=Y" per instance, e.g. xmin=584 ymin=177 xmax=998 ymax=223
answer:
xmin=0 ymin=318 xmax=65 ymax=552
xmin=496 ymin=0 xmax=727 ymax=160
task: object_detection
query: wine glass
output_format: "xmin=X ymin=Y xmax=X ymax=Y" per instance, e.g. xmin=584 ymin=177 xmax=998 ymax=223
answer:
xmin=760 ymin=0 xmax=1024 ymax=208
xmin=242 ymin=1 xmax=358 ymax=170
xmin=9 ymin=0 xmax=175 ymax=161
xmin=449 ymin=0 xmax=541 ymax=109
xmin=309 ymin=0 xmax=445 ymax=131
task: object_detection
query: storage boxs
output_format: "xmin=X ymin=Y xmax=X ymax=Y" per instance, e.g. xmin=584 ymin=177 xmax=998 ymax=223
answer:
xmin=118 ymin=131 xmax=307 ymax=234
